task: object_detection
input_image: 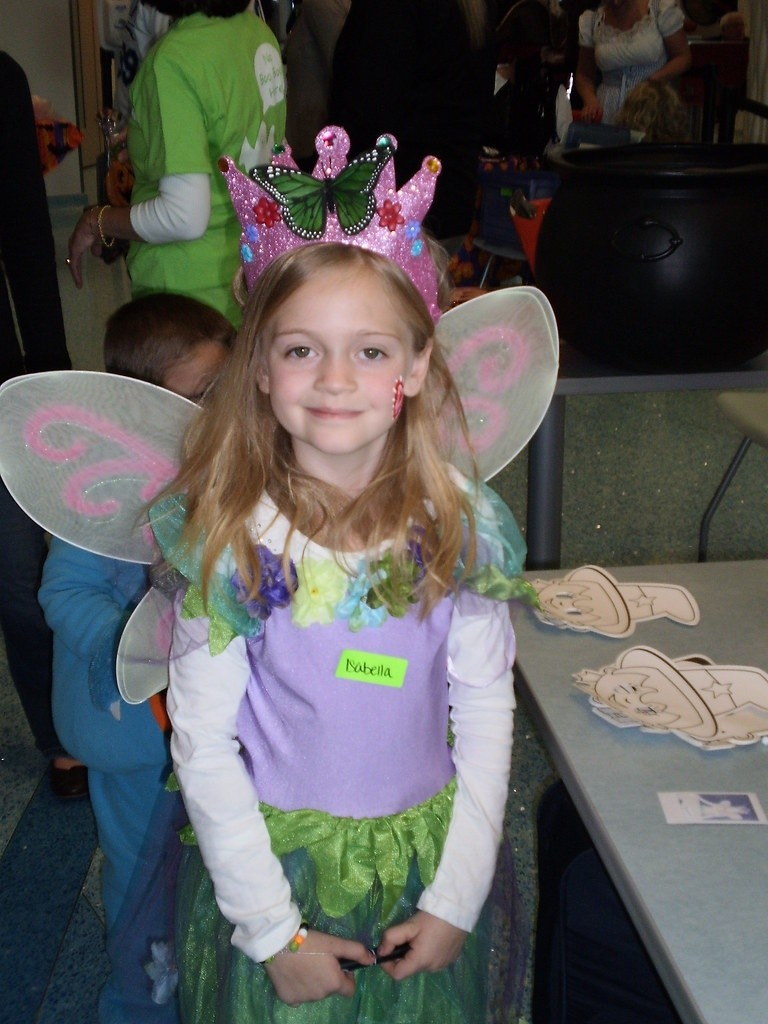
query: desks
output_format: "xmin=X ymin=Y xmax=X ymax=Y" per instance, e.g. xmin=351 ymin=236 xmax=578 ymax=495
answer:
xmin=523 ymin=335 xmax=767 ymax=570
xmin=507 ymin=561 xmax=767 ymax=1023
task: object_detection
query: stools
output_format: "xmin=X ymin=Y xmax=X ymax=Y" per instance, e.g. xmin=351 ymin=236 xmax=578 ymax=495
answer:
xmin=697 ymin=391 xmax=767 ymax=563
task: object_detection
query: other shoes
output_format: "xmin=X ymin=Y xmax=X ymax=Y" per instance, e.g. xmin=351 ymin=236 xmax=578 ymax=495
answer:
xmin=51 ymin=765 xmax=89 ymax=798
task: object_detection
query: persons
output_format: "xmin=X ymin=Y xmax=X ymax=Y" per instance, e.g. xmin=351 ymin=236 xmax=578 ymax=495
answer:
xmin=571 ymin=0 xmax=698 ymax=131
xmin=38 ymin=291 xmax=248 ymax=1024
xmin=164 ymin=128 xmax=530 ymax=1023
xmin=66 ymin=1 xmax=289 ymax=335
xmin=1 ymin=47 xmax=95 ymax=808
